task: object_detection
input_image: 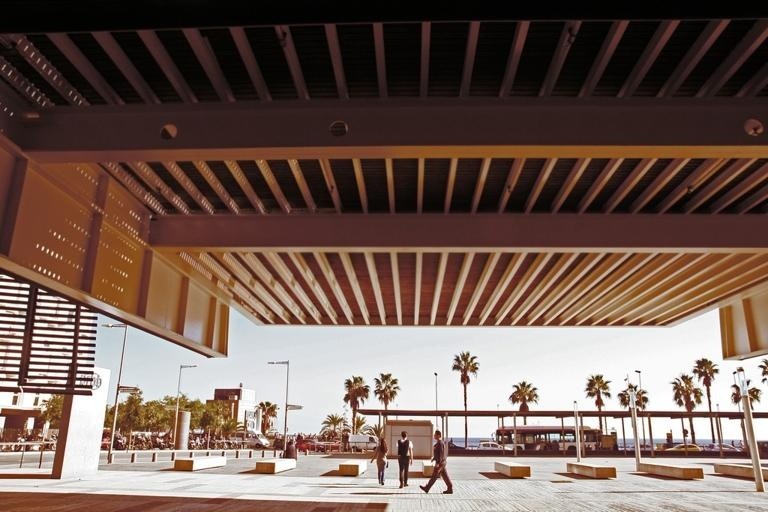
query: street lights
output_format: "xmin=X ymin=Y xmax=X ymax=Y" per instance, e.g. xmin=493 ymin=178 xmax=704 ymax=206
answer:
xmin=102 ymin=323 xmax=128 ymax=462
xmin=172 ymin=365 xmax=197 ymax=445
xmin=268 ymin=361 xmax=290 ymax=449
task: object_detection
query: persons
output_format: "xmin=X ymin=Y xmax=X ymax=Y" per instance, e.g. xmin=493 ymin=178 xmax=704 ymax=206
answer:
xmin=738 ymin=439 xmax=743 ymax=446
xmin=369 ymin=437 xmax=389 ymax=485
xmin=418 ymin=430 xmax=453 ymax=494
xmin=396 ymin=430 xmax=414 ymax=488
xmin=285 ymin=439 xmax=298 ymax=458
xmin=732 ymin=440 xmax=735 ymax=446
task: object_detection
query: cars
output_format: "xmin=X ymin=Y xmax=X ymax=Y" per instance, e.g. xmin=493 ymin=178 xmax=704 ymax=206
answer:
xmin=666 ymin=444 xmax=700 ymax=451
xmin=295 ymin=439 xmax=324 ymax=452
xmin=448 ymin=443 xmax=464 ymax=449
xmin=709 ymin=442 xmax=739 ymax=451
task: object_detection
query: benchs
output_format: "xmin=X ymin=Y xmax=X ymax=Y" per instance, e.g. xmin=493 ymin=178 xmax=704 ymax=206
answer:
xmin=494 ymin=461 xmax=532 ymax=479
xmin=421 ymin=460 xmax=438 ymax=476
xmin=254 ymin=459 xmax=298 ymax=474
xmin=713 ymin=463 xmax=768 ymax=481
xmin=338 ymin=461 xmax=370 ymax=476
xmin=173 ymin=455 xmax=229 ymax=472
xmin=566 ymin=463 xmax=617 ymax=479
xmin=635 ymin=463 xmax=706 ymax=481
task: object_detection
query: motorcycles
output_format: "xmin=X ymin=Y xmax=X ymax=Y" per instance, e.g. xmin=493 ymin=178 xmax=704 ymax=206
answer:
xmin=188 ymin=436 xmax=227 ymax=450
xmin=116 ymin=432 xmax=174 ymax=450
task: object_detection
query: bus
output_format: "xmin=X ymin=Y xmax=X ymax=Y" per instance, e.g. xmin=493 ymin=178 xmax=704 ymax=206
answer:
xmin=495 ymin=425 xmax=597 ymax=459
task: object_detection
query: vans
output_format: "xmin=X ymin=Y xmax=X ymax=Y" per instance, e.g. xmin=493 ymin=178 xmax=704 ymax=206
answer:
xmin=477 ymin=442 xmax=503 ymax=450
xmin=348 ymin=435 xmax=380 ymax=451
xmin=230 ymin=430 xmax=270 ymax=448
